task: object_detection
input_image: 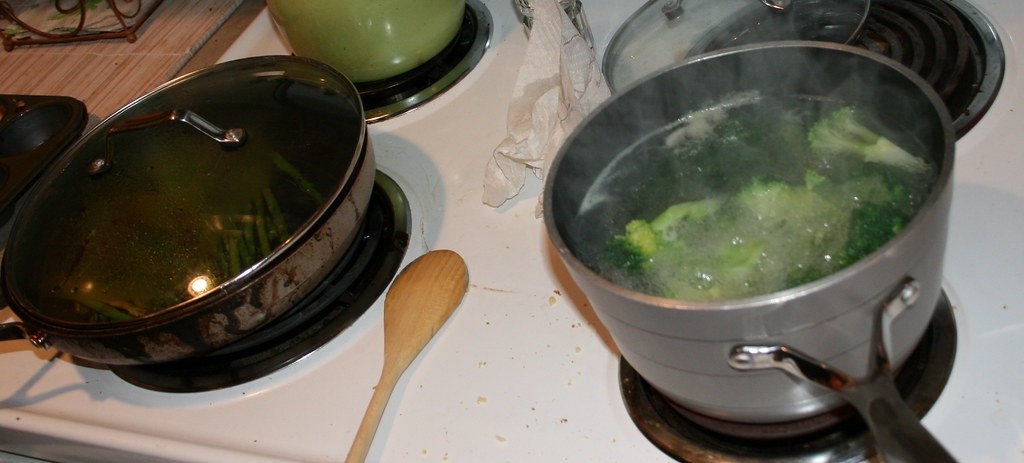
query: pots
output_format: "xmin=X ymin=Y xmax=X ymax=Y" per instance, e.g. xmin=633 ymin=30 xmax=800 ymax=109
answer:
xmin=0 ymin=52 xmax=377 ymax=369
xmin=264 ymin=0 xmax=468 ymax=85
xmin=543 ymin=41 xmax=961 ymax=463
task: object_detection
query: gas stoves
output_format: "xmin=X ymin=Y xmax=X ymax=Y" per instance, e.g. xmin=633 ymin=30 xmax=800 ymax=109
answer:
xmin=0 ymin=0 xmax=1024 ymax=463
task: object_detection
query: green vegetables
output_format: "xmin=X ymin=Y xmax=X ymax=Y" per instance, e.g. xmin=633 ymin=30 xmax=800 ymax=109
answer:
xmin=72 ymin=138 xmax=334 ymax=320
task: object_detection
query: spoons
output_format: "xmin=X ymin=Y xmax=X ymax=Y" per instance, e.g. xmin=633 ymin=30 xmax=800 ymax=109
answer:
xmin=344 ymin=250 xmax=467 ymax=463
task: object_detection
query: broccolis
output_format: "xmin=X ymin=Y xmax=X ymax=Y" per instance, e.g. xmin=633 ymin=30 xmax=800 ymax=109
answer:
xmin=599 ymin=101 xmax=934 ymax=303
